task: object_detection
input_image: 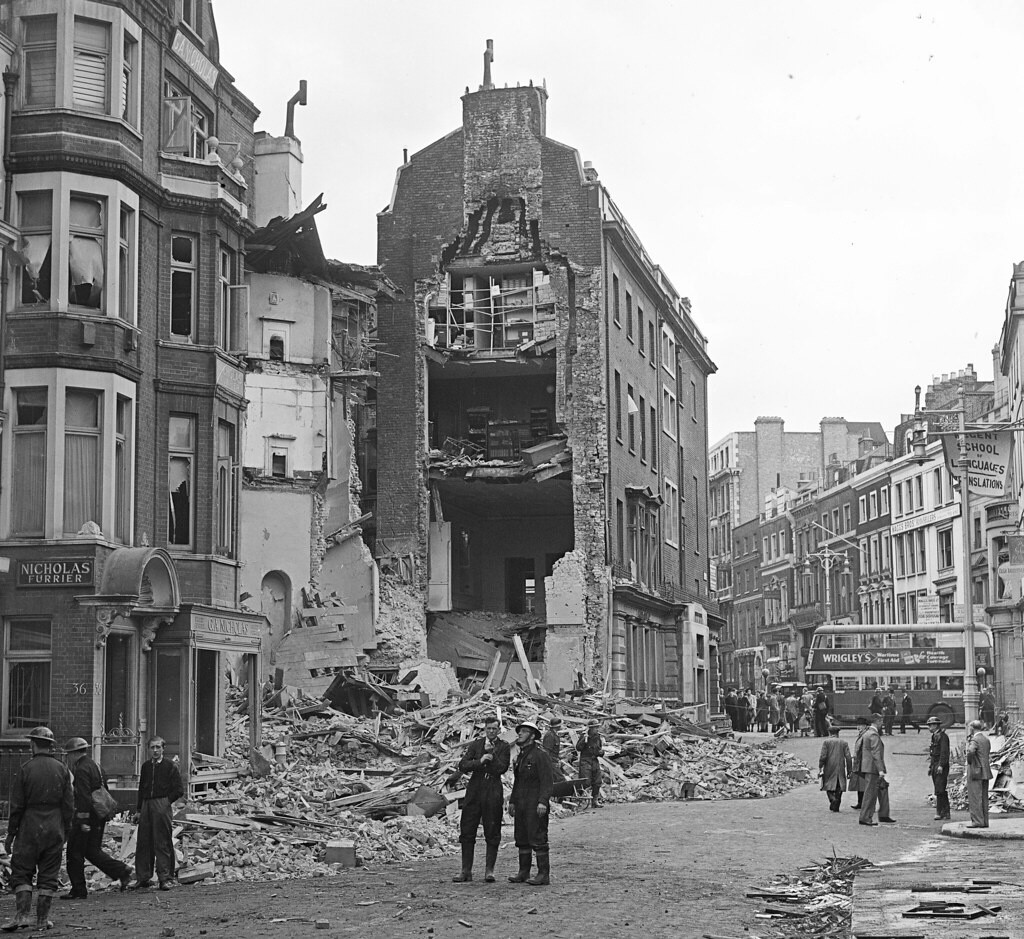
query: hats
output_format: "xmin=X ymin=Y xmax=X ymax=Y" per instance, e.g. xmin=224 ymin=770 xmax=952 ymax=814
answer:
xmin=857 ymin=718 xmax=869 ymax=725
xmin=827 ymin=726 xmax=839 ymax=731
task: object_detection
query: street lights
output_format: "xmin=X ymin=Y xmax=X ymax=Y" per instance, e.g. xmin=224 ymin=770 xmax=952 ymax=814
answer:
xmin=906 ymin=381 xmax=981 ymax=796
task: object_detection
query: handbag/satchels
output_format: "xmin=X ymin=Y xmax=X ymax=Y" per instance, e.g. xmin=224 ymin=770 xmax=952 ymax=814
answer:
xmin=818 ymin=702 xmax=826 ymax=710
xmin=89 ymin=786 xmax=117 ymax=820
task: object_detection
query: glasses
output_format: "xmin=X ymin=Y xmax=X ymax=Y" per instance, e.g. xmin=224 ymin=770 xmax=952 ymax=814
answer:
xmin=518 ymin=728 xmax=532 ymax=733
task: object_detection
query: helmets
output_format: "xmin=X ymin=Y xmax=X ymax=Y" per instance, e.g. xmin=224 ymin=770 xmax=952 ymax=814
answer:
xmin=588 ymin=719 xmax=602 ymax=727
xmin=515 ymin=722 xmax=541 ymax=740
xmin=25 ymin=727 xmax=57 ymax=743
xmin=927 ymin=716 xmax=942 ymax=724
xmin=65 ymin=737 xmax=91 ymax=752
xmin=549 ymin=718 xmax=564 ymax=728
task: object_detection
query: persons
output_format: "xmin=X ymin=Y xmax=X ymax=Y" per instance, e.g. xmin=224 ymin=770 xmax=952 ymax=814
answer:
xmin=926 ymin=717 xmax=951 ymax=820
xmin=965 ymin=721 xmax=994 ymax=828
xmin=452 ymin=717 xmax=553 ymax=884
xmin=0 ymin=726 xmax=184 ymax=931
xmin=542 ymin=716 xmax=606 ymax=808
xmin=817 ymin=713 xmax=897 ymax=825
xmin=868 ymin=687 xmax=921 ymax=736
xmin=724 ymin=684 xmax=834 ymax=737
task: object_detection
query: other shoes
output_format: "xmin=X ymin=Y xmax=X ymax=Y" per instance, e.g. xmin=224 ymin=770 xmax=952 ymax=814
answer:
xmin=830 ymin=800 xmax=839 ymax=812
xmin=160 ymin=881 xmax=173 ymax=890
xmin=859 ymin=821 xmax=878 ymax=825
xmin=119 ymin=865 xmax=132 ymax=891
xmin=967 ymin=824 xmax=988 ymax=828
xmin=131 ymin=881 xmax=146 ymax=890
xmin=879 ymin=817 xmax=896 ymax=822
xmin=851 ymin=804 xmax=861 ymax=809
xmin=59 ymin=891 xmax=87 ymax=899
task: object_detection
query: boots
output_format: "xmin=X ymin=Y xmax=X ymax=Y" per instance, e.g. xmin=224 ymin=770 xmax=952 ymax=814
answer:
xmin=508 ymin=848 xmax=532 ymax=882
xmin=1 ymin=885 xmax=32 ymax=931
xmin=453 ymin=844 xmax=474 ymax=882
xmin=486 ymin=844 xmax=497 ymax=881
xmin=934 ymin=792 xmax=951 ymax=820
xmin=37 ymin=889 xmax=53 ymax=931
xmin=528 ymin=850 xmax=549 ymax=885
xmin=592 ymin=794 xmax=603 ymax=808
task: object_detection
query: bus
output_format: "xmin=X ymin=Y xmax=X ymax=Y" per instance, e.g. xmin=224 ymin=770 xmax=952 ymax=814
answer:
xmin=806 ymin=621 xmax=996 ymax=730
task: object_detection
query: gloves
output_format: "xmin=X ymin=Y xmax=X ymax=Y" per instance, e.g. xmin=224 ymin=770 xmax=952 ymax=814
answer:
xmin=64 ymin=822 xmax=72 ymax=843
xmin=5 ymin=835 xmax=13 ymax=854
